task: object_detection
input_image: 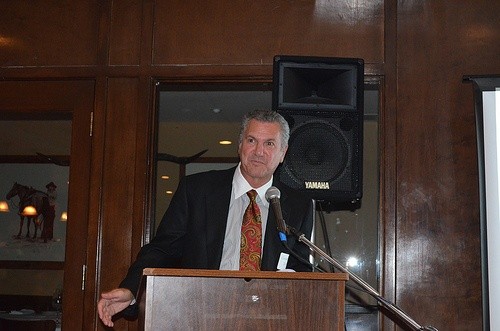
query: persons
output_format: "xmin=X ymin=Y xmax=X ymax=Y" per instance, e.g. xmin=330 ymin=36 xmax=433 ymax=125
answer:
xmin=96 ymin=111 xmax=315 ymax=328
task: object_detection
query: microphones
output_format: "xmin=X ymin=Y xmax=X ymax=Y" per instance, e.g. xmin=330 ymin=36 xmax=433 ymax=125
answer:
xmin=266 ymin=186 xmax=287 ymax=242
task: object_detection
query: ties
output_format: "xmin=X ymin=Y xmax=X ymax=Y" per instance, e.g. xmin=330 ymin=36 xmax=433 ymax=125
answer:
xmin=239 ymin=189 xmax=262 ymax=271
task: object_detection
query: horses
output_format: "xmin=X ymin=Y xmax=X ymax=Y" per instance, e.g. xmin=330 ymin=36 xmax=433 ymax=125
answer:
xmin=6 ymin=181 xmax=48 ymax=241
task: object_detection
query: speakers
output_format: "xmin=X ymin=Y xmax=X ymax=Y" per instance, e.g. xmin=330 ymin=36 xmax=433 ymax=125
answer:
xmin=272 ymin=56 xmax=362 ymax=206
